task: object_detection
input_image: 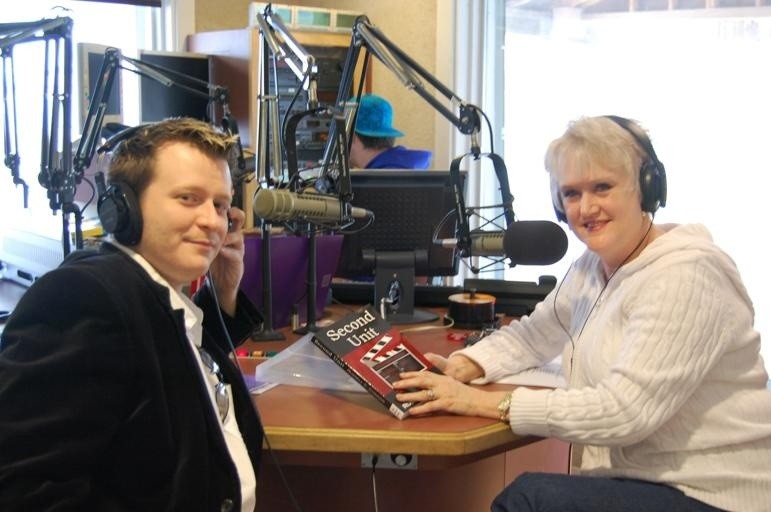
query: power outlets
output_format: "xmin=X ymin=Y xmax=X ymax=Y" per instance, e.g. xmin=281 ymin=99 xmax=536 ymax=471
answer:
xmin=361 ymin=450 xmax=417 ymax=469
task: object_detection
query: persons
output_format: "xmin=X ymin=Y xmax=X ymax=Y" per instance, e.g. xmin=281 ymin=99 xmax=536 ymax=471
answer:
xmin=392 ymin=114 xmax=771 ymax=511
xmin=1 ymin=114 xmax=268 ymax=511
xmin=343 ymin=94 xmax=434 ymax=286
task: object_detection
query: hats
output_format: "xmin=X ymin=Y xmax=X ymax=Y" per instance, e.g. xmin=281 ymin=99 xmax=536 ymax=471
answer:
xmin=344 ymin=96 xmax=404 ymax=140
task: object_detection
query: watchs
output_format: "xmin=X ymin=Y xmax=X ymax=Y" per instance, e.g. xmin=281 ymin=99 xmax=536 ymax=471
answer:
xmin=497 ymin=390 xmax=512 ymax=422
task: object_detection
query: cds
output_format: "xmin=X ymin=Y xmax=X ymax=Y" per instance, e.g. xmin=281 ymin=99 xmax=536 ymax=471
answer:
xmin=448 ymin=292 xmax=497 ymax=328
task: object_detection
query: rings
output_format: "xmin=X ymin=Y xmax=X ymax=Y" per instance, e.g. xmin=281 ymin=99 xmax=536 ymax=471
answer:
xmin=428 ymin=389 xmax=435 ymax=401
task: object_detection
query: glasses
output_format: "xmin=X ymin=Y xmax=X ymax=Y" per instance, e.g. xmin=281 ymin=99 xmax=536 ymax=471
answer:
xmin=195 ymin=346 xmax=230 ymax=423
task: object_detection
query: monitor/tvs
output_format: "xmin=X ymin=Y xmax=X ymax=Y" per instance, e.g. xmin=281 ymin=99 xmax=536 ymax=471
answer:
xmin=337 ymin=169 xmax=471 ymax=326
xmin=76 ymin=41 xmax=125 ymax=136
xmin=137 ymin=49 xmax=216 ymax=126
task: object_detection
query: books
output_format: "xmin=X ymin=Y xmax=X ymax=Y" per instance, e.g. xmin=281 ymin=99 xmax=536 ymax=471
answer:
xmin=310 ymin=304 xmax=446 ymax=420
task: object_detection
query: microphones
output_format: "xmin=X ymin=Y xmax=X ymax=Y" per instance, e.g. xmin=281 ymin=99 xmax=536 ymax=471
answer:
xmin=433 ymin=221 xmax=568 ymax=264
xmin=252 ymin=189 xmax=374 ymax=225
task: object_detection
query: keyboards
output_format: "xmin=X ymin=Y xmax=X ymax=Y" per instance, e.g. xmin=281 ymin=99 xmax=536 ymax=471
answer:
xmin=331 ymin=281 xmax=462 ymax=306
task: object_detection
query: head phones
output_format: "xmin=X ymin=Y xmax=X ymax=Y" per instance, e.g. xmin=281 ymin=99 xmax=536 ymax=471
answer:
xmin=552 ymin=115 xmax=668 ymax=224
xmin=96 ymin=115 xmax=234 ymax=247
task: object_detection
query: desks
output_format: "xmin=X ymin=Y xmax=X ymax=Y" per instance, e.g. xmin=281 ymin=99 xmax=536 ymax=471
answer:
xmin=231 ymin=304 xmax=577 ymax=512
